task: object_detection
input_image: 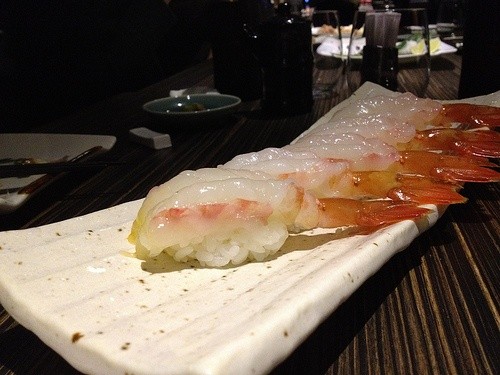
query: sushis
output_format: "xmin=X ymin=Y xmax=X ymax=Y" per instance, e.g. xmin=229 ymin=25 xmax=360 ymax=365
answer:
xmin=126 ymin=91 xmax=500 ymax=267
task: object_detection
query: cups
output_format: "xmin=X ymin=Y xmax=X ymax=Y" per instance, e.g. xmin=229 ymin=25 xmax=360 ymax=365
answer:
xmin=292 ymin=11 xmax=343 ymax=92
xmin=346 ymin=8 xmax=429 ymax=96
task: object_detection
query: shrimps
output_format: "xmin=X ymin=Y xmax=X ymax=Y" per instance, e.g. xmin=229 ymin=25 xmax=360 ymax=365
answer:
xmin=127 ymin=91 xmax=500 ymax=269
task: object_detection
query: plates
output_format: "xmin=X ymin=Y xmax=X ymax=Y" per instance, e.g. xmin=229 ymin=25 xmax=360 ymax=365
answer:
xmin=316 ymin=37 xmax=458 ymax=61
xmin=0 ymin=80 xmax=500 ymax=375
xmin=142 ymin=93 xmax=241 ymax=125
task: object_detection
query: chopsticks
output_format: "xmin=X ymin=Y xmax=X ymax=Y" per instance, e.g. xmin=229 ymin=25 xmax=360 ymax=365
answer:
xmin=0 ymin=151 xmax=122 ymax=171
